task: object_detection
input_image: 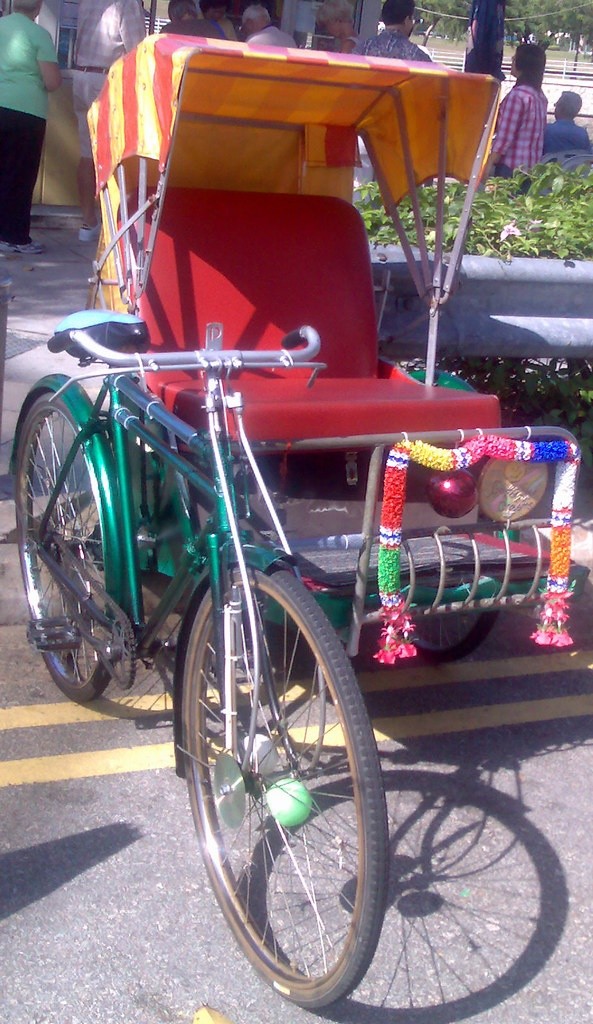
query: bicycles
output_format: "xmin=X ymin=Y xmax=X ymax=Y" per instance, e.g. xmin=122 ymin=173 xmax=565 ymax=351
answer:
xmin=5 ymin=310 xmax=389 ymax=1010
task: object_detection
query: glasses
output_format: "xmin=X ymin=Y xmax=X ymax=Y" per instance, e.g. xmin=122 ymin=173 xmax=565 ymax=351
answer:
xmin=237 ymin=20 xmax=249 ymax=31
xmin=512 ymin=55 xmax=517 ymax=61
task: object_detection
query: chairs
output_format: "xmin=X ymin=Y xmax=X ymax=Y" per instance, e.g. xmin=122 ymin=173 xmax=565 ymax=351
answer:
xmin=532 ymin=149 xmax=593 ymax=195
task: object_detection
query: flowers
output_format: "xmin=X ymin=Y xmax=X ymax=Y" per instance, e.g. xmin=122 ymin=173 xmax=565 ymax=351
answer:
xmin=373 ymin=435 xmax=577 ymax=664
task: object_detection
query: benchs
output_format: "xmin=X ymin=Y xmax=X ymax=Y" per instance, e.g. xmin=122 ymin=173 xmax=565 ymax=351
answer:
xmin=117 ymin=185 xmax=503 ymax=460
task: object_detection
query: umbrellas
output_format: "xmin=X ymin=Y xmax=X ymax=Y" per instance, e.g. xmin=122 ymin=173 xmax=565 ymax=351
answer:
xmin=463 ymin=0 xmax=506 ymax=82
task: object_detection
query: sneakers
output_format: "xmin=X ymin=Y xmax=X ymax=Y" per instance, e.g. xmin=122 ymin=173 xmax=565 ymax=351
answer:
xmin=0 ymin=240 xmax=44 ymax=253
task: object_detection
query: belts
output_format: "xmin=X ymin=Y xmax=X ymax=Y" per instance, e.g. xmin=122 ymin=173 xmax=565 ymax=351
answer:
xmin=75 ymin=66 xmax=109 ymax=74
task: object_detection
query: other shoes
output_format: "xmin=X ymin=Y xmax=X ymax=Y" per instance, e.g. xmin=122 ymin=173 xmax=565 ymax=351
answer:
xmin=79 ymin=219 xmax=104 ymax=244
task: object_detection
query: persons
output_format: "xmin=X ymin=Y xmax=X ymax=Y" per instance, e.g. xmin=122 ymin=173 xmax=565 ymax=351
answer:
xmin=542 ymin=89 xmax=591 ymax=170
xmin=73 ymin=0 xmax=148 ymax=242
xmin=143 ymin=0 xmax=433 ymax=62
xmin=486 ymin=43 xmax=548 ymax=198
xmin=0 ymin=0 xmax=63 ymax=255
